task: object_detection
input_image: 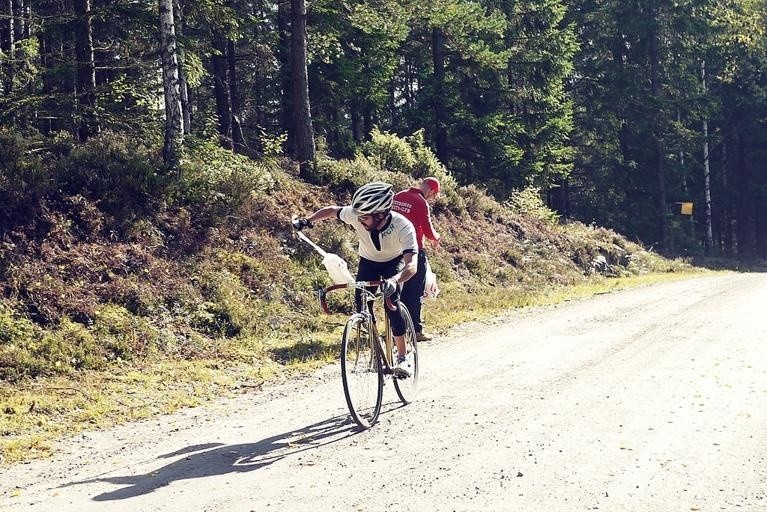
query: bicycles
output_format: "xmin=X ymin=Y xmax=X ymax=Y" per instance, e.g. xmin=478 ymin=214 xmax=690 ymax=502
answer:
xmin=318 ymin=280 xmax=419 ymax=430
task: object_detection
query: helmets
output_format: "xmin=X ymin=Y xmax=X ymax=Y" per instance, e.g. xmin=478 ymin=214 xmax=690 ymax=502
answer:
xmin=351 ymin=182 xmax=395 ymax=216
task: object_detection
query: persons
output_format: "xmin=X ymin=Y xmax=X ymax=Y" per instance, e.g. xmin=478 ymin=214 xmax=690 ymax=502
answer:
xmin=391 ymin=176 xmax=440 ymax=341
xmin=293 ymin=182 xmax=419 ymax=377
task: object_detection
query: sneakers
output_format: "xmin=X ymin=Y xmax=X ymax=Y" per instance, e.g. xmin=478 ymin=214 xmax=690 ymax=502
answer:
xmin=394 ymin=354 xmax=412 ymax=378
xmin=416 ymin=332 xmax=431 ymax=341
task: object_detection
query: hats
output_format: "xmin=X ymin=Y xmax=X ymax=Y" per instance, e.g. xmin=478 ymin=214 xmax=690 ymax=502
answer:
xmin=423 ymin=176 xmax=439 ymax=193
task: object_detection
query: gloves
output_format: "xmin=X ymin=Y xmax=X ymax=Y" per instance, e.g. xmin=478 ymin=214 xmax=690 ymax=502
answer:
xmin=382 ymin=278 xmax=397 ymax=299
